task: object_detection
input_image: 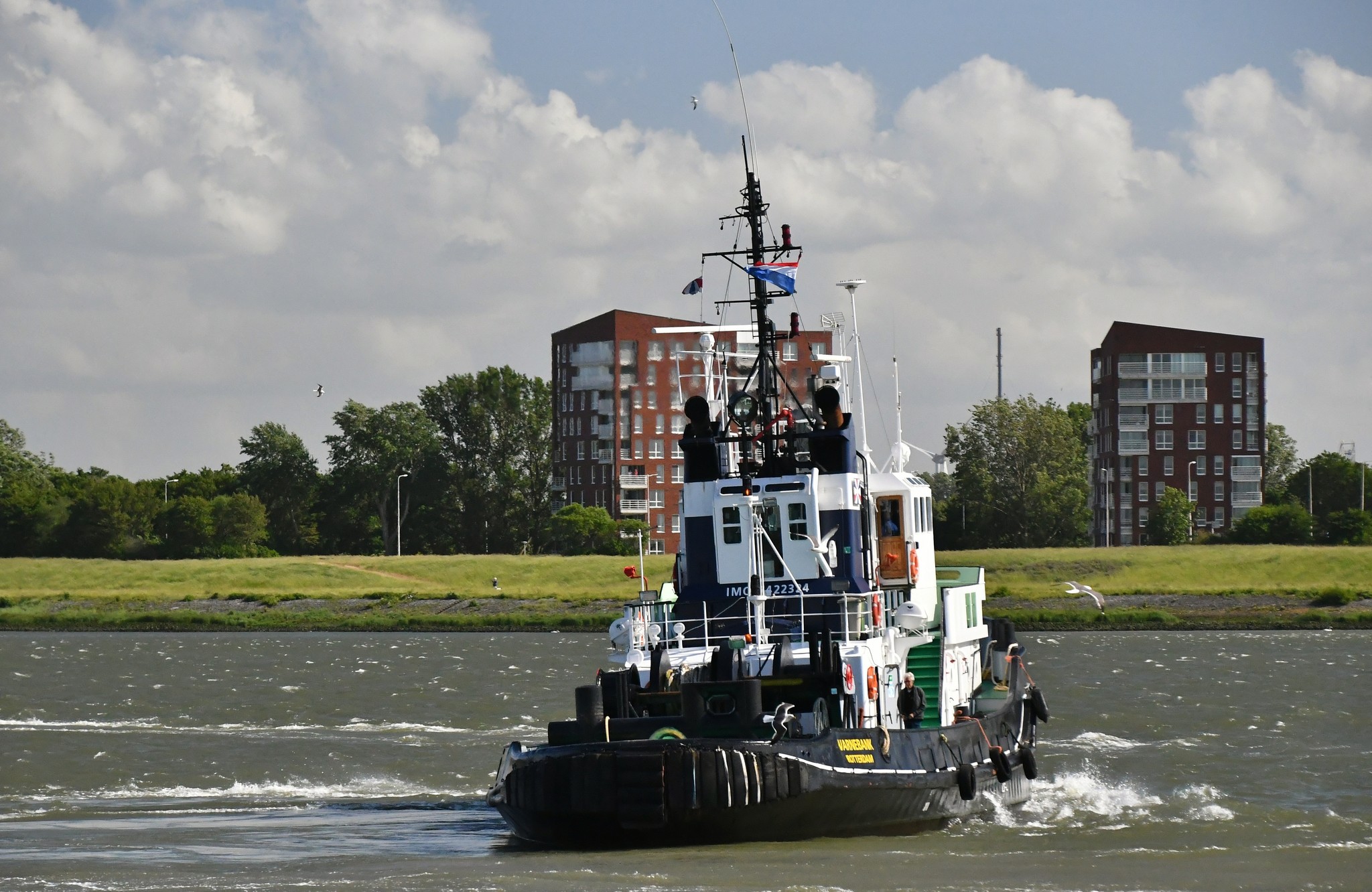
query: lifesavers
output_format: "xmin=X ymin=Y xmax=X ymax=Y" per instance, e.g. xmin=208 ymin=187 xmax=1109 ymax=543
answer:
xmin=910 ymin=549 xmax=919 ymax=575
xmin=911 ymin=571 xmax=919 ymax=583
xmin=988 ymin=747 xmax=1012 ymax=783
xmin=872 ymin=594 xmax=882 ymax=629
xmin=868 ymin=666 xmax=879 ymax=702
xmin=958 ymin=763 xmax=976 ymax=801
xmin=1020 ymin=748 xmax=1038 ymax=780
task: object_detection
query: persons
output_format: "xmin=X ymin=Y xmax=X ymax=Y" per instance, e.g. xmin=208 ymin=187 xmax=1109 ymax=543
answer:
xmin=897 ymin=671 xmax=927 ymax=728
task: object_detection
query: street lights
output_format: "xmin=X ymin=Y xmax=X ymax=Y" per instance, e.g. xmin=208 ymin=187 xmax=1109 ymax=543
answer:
xmin=1304 ymin=463 xmax=1314 ymax=537
xmin=1100 ymin=468 xmax=1109 ymax=549
xmin=165 ymin=479 xmax=180 ymax=538
xmin=1188 ymin=460 xmax=1197 ymax=542
xmin=397 ymin=474 xmax=408 ymax=557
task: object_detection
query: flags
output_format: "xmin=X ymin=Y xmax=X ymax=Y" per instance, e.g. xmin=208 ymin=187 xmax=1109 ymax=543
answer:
xmin=681 ymin=276 xmax=703 ymax=296
xmin=743 ymin=261 xmax=798 ymax=294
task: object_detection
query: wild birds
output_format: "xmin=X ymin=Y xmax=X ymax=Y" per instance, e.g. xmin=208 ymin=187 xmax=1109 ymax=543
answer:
xmin=786 ymin=523 xmax=840 ymax=555
xmin=313 ymin=383 xmax=326 ymax=398
xmin=690 ymin=95 xmax=699 ymax=111
xmin=762 ymin=701 xmax=798 ymax=745
xmin=1048 ymin=579 xmax=1106 ymax=616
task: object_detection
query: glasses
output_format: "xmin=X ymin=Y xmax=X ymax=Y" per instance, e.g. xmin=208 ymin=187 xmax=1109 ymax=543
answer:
xmin=905 ymin=680 xmax=912 ymax=683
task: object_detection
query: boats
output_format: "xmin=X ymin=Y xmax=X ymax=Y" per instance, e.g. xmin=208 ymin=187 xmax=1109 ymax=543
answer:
xmin=486 ymin=134 xmax=1051 ymax=846
